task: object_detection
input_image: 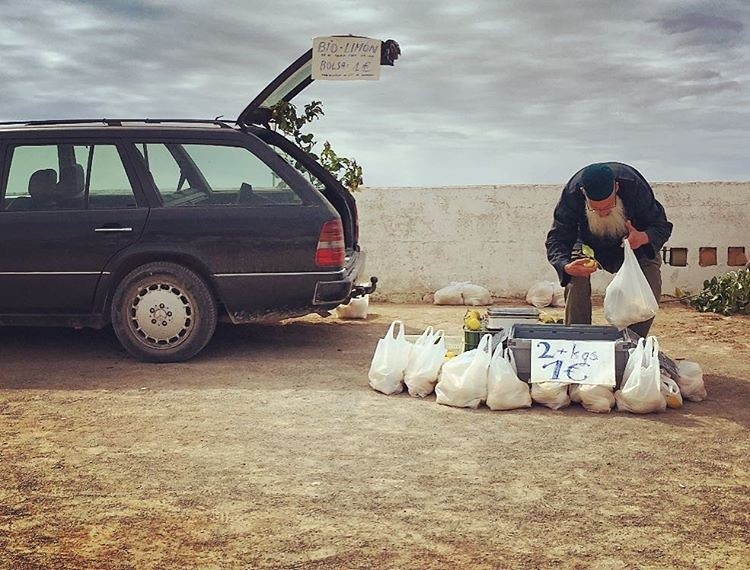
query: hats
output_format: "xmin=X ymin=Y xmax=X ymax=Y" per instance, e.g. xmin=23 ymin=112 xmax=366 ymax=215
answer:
xmin=581 ymin=164 xmax=615 ymax=200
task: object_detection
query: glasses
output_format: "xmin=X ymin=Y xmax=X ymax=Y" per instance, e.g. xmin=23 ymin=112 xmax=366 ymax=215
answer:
xmin=586 ymin=188 xmax=616 ymax=212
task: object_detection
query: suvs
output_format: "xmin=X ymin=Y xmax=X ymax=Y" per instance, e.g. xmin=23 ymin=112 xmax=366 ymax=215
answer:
xmin=1 ymin=34 xmax=402 ymax=363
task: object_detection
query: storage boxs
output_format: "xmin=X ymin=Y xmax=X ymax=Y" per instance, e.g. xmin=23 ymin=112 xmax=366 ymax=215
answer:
xmin=506 ymin=322 xmax=627 ymax=391
xmin=463 ymin=318 xmax=507 ymax=354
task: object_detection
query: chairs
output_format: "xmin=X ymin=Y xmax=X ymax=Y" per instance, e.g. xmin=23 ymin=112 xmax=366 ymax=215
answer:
xmin=56 ymin=164 xmax=86 ymax=210
xmin=6 ymin=168 xmax=62 ymax=211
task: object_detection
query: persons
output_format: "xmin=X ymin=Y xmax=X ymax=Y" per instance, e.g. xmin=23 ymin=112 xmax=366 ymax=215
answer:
xmin=545 ymin=162 xmax=673 ymax=338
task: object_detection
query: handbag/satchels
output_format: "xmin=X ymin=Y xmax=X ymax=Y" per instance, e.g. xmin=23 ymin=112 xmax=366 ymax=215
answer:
xmin=434 ymin=281 xmax=492 ymax=306
xmin=335 ymin=280 xmax=370 ymax=319
xmin=615 ymin=337 xmax=666 ymax=412
xmin=529 ymin=380 xmax=570 ymax=410
xmin=673 ymin=359 xmax=707 ymax=402
xmin=434 ymin=334 xmax=491 ymax=410
xmin=569 ymin=382 xmax=615 ymax=415
xmin=486 ymin=343 xmax=532 ymax=410
xmin=526 ymin=280 xmax=565 ymax=308
xmin=658 ymin=372 xmax=684 ymax=407
xmin=404 ymin=325 xmax=445 ymax=398
xmin=368 ymin=321 xmax=414 ymax=396
xmin=603 ymin=239 xmax=658 ymax=331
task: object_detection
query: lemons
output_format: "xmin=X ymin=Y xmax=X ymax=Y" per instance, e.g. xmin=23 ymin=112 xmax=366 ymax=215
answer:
xmin=464 ymin=310 xmax=480 ymax=331
xmin=445 ymin=352 xmax=456 ymax=357
